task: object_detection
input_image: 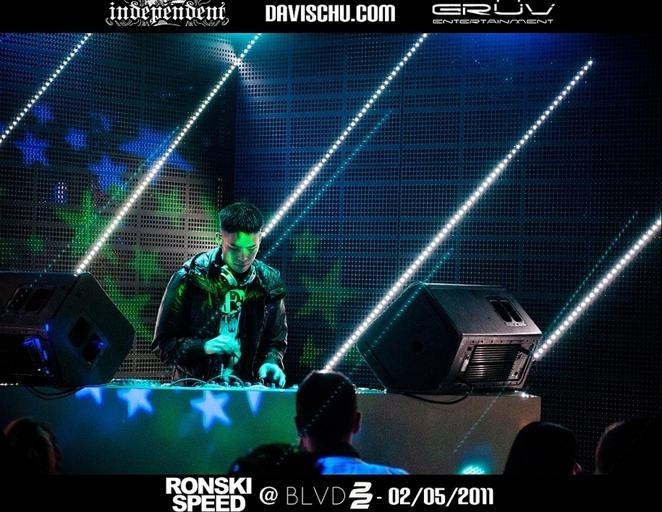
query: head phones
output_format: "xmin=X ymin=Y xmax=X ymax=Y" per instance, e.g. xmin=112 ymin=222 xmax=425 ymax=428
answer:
xmin=217 ymin=262 xmax=240 ymax=291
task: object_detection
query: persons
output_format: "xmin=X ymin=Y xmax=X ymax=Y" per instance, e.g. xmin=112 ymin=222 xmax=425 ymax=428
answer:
xmin=503 ymin=421 xmax=582 ymax=498
xmin=294 ymin=372 xmax=410 ymax=475
xmin=595 ymin=418 xmax=661 ymax=475
xmin=5 ymin=416 xmax=64 ymax=476
xmin=228 ymin=442 xmax=324 ymax=476
xmin=150 ymin=200 xmax=288 ymax=389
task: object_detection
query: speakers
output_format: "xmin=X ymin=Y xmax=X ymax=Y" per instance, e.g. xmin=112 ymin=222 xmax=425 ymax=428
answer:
xmin=0 ymin=270 xmax=136 ymax=389
xmin=355 ymin=280 xmax=543 ymax=396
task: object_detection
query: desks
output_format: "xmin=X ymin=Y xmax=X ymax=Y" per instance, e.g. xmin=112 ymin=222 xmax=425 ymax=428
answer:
xmin=1 ymin=376 xmax=542 ymax=476
